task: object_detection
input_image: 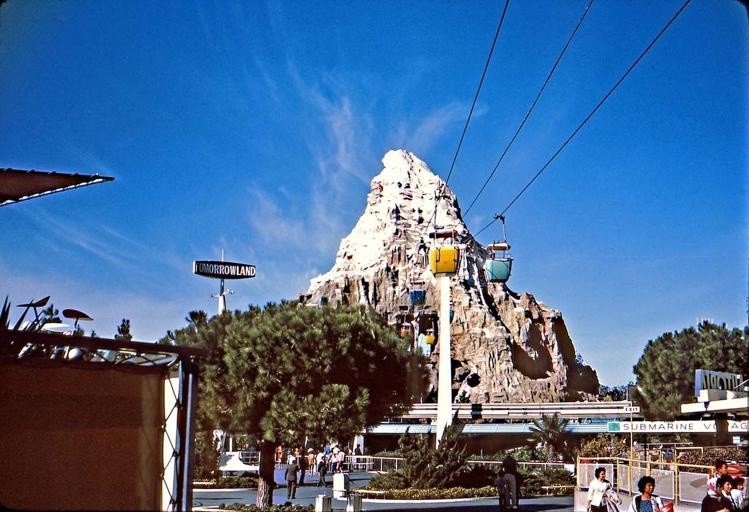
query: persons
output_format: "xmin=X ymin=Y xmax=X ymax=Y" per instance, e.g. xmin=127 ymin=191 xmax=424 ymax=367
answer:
xmin=585 ymin=467 xmax=613 ymax=512
xmin=494 ymin=452 xmax=523 ymax=512
xmin=284 ymin=460 xmax=300 ymax=499
xmin=276 ymin=439 xmax=363 ymax=488
xmin=626 ymin=475 xmax=666 ymax=512
xmin=700 ymin=459 xmax=748 ymax=512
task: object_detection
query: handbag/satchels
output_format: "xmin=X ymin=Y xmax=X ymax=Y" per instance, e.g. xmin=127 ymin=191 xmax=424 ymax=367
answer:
xmin=657 ymin=502 xmax=674 ymax=512
